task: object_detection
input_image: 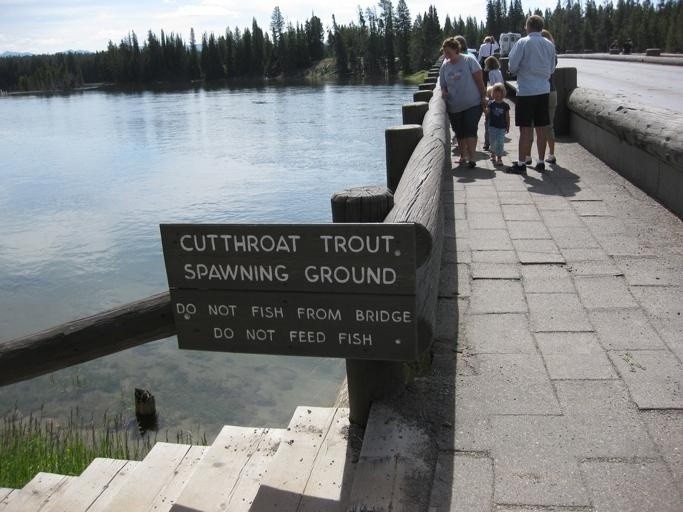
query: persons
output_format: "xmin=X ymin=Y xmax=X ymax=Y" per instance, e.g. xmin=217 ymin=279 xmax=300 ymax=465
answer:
xmin=437 ymin=35 xmax=504 ymax=152
xmin=484 ymin=82 xmax=510 ymax=167
xmin=503 ymin=14 xmax=557 ymax=174
xmin=524 ymin=28 xmax=558 ymax=166
xmin=437 ymin=36 xmax=486 ymax=169
xmin=608 ymin=38 xmax=633 ymax=55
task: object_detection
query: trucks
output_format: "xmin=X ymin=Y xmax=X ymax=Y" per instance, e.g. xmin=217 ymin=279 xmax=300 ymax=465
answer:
xmin=498 ymin=31 xmax=523 ymax=54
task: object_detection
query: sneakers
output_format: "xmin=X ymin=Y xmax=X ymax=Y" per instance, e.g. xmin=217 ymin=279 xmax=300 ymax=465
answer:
xmin=489 ymin=153 xmax=505 ymax=166
xmin=545 ymin=157 xmax=557 ymax=165
xmin=458 ymin=157 xmax=476 ymax=168
xmin=527 ymin=161 xmax=546 ymax=173
xmin=505 ymin=155 xmax=532 ymax=175
